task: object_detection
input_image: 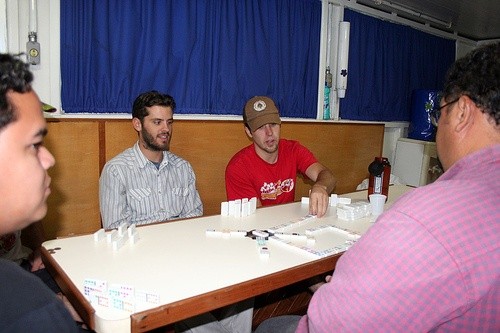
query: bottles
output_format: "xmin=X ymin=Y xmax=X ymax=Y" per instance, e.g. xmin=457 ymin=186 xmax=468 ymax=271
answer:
xmin=26 ymin=35 xmax=41 ymax=65
xmin=323 ymin=82 xmax=330 ymax=120
xmin=381 ymin=157 xmax=391 ymax=203
xmin=325 ymin=69 xmax=332 ymax=88
xmin=368 ymin=156 xmax=384 ymax=201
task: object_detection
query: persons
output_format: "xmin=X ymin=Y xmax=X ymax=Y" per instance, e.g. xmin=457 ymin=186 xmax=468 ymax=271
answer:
xmin=225 ymin=97 xmax=336 ymax=291
xmin=98 ymin=90 xmax=253 ymax=333
xmin=255 ymin=42 xmax=500 ymax=333
xmin=0 ymin=54 xmax=80 ymax=333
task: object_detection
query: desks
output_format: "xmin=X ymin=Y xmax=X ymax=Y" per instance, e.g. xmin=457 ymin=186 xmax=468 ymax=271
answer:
xmin=38 ymin=184 xmax=419 ymax=333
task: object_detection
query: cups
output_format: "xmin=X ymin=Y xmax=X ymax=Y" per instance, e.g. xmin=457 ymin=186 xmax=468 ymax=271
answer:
xmin=369 ymin=194 xmax=387 ymax=216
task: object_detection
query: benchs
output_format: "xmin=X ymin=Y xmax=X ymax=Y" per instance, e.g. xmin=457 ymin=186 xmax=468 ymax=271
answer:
xmin=22 ymin=119 xmax=385 ymax=327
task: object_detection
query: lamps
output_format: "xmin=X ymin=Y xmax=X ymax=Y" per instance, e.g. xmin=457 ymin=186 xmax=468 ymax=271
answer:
xmin=375 ymin=0 xmax=451 ymax=28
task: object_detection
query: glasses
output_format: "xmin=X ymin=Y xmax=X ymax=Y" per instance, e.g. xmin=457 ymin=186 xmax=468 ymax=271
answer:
xmin=430 ymin=97 xmax=460 ymax=127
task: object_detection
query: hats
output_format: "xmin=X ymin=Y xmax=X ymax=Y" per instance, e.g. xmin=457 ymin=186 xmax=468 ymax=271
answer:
xmin=243 ymin=95 xmax=281 ymax=132
